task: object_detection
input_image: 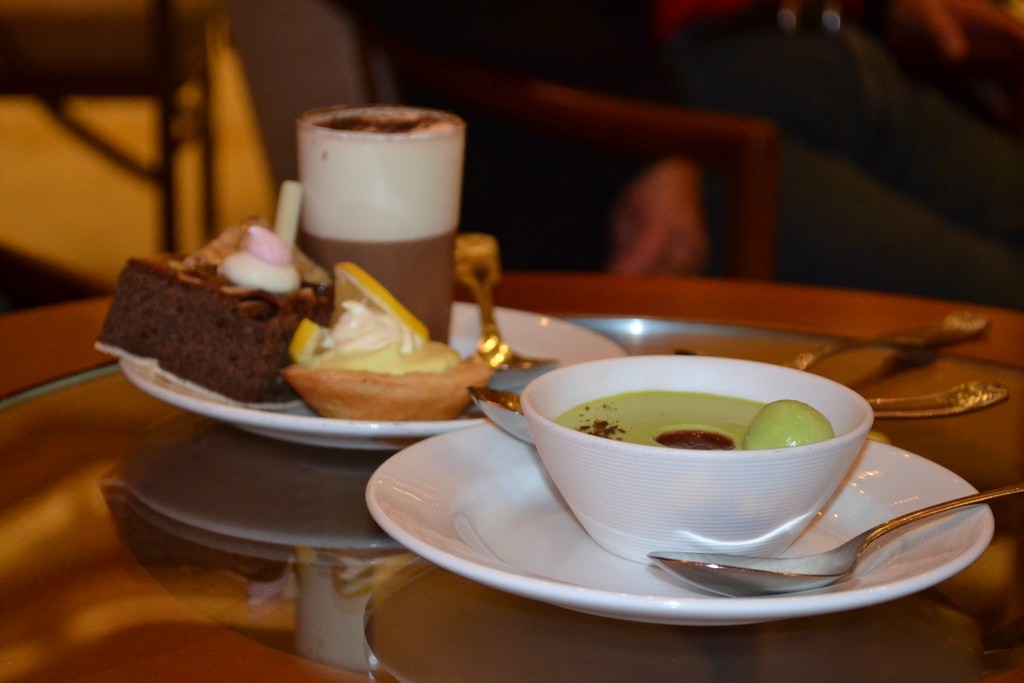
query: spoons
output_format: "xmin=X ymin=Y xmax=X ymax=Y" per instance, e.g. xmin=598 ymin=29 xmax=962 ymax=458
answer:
xmin=468 ymin=379 xmax=1010 ymax=445
xmin=648 ymin=481 xmax=1024 ymax=598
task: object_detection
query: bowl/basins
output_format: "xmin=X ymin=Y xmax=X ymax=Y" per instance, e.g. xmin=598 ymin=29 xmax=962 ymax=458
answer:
xmin=521 ymin=355 xmax=873 ymax=564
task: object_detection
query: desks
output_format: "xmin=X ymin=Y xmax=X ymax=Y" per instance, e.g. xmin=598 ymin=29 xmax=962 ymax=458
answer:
xmin=0 ymin=267 xmax=1024 ymax=683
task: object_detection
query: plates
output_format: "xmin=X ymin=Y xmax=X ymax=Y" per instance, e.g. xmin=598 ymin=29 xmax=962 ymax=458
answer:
xmin=366 ymin=422 xmax=995 ymax=626
xmin=120 ymin=301 xmax=627 ymax=449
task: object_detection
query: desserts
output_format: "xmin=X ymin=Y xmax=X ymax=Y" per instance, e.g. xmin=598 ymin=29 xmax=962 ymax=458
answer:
xmin=98 ymin=223 xmax=335 ymax=403
xmin=280 ymin=261 xmax=492 ymax=421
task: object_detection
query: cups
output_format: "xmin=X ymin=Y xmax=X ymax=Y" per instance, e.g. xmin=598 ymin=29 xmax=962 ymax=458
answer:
xmin=294 ymin=105 xmax=465 ymax=345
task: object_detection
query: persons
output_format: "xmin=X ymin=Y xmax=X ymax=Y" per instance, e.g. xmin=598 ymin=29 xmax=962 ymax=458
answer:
xmin=329 ymin=1 xmax=1024 ymax=312
